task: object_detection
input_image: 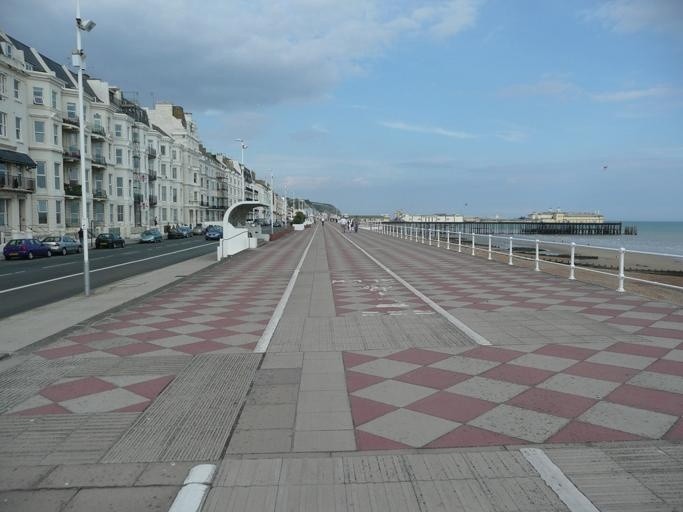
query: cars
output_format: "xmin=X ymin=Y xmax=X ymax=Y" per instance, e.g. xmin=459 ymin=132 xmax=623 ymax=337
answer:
xmin=245 ymin=218 xmax=281 ymax=228
xmin=3 ymin=223 xmax=223 ymax=261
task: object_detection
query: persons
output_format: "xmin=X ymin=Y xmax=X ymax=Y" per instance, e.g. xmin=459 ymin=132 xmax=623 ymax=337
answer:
xmin=335 ymin=214 xmax=359 ymax=234
xmin=77 ymin=227 xmax=83 ymax=244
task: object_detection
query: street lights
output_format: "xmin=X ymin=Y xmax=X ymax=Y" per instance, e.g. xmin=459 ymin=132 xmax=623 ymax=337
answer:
xmin=74 ymin=16 xmax=98 ymax=299
xmin=230 ymin=137 xmax=245 ymax=204
